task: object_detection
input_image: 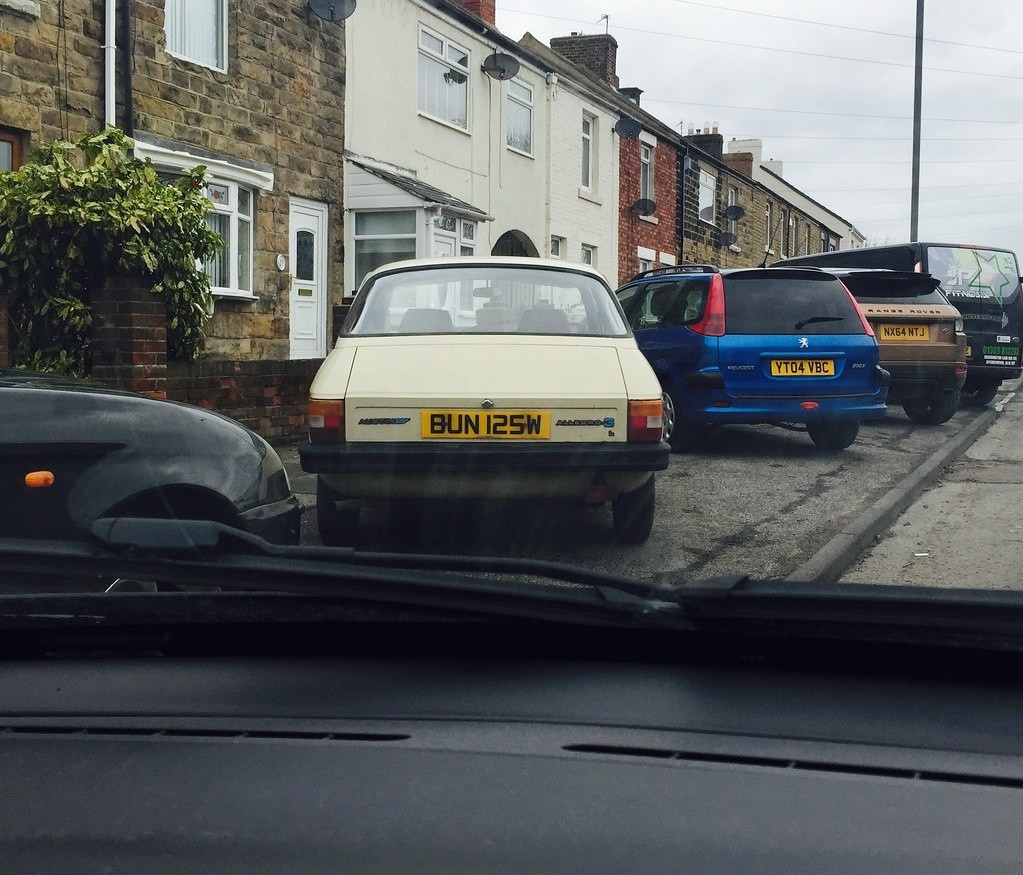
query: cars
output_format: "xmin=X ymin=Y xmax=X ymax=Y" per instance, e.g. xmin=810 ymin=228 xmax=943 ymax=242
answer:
xmin=298 ymin=254 xmax=673 ymax=546
xmin=0 ymin=364 xmax=309 ymax=545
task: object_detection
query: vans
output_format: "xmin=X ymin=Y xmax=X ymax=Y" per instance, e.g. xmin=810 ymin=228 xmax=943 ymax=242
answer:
xmin=764 ymin=241 xmax=1023 ymax=407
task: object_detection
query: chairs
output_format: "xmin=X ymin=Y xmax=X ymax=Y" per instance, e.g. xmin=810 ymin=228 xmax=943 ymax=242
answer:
xmin=519 ymin=308 xmax=571 ymax=331
xmin=399 ymin=309 xmax=454 ymax=331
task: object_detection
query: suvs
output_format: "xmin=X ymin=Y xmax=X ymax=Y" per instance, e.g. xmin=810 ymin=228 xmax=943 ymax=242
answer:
xmin=762 ymin=267 xmax=968 ymax=426
xmin=603 ymin=263 xmax=894 ymax=451
